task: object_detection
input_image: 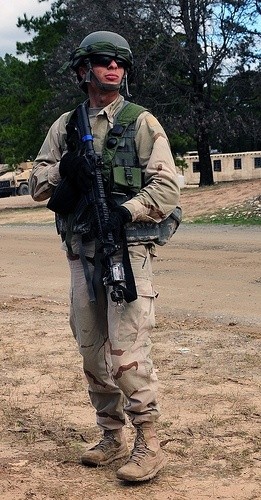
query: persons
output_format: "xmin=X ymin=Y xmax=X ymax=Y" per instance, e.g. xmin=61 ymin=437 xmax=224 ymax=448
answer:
xmin=30 ymin=31 xmax=179 ymax=481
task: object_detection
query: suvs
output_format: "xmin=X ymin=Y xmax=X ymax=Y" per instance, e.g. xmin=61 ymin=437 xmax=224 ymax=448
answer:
xmin=0 ymin=162 xmax=34 ymax=196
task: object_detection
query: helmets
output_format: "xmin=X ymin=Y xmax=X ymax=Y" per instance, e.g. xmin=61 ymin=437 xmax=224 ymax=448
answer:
xmin=70 ymin=31 xmax=132 ymax=71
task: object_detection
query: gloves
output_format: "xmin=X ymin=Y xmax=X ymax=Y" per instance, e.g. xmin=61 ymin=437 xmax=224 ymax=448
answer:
xmin=58 ymin=155 xmax=85 ymax=181
xmin=100 ymin=210 xmax=128 ymax=242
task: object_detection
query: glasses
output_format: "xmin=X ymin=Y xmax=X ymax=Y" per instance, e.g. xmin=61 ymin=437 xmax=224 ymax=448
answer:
xmin=89 ymin=55 xmax=126 ymax=68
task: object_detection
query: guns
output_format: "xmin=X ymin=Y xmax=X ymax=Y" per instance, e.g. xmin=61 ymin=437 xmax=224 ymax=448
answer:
xmin=67 ymin=103 xmax=125 ymax=315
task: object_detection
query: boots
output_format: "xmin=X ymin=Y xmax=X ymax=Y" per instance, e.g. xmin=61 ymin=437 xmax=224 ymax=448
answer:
xmin=116 ymin=423 xmax=166 ymax=483
xmin=81 ymin=429 xmax=128 ymax=466
